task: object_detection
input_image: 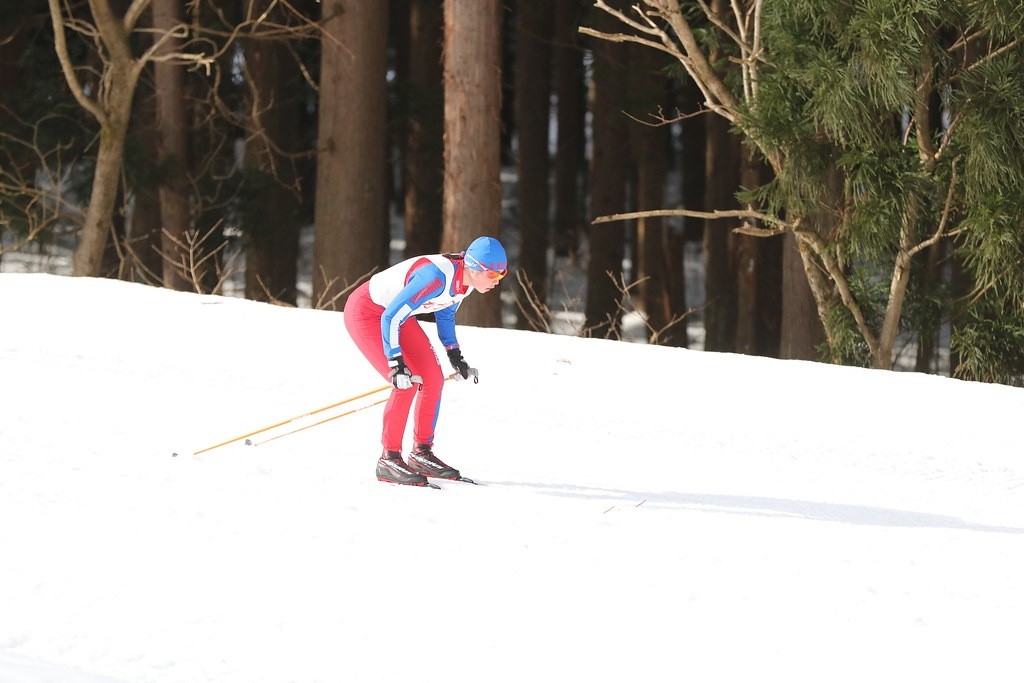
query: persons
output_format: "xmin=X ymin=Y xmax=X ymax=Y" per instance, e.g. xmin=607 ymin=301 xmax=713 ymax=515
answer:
xmin=344 ymin=236 xmax=508 ymax=487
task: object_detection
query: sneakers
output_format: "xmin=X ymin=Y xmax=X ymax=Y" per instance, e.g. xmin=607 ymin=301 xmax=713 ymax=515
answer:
xmin=409 ymin=450 xmax=460 ymax=478
xmin=375 ymin=457 xmax=427 ymax=486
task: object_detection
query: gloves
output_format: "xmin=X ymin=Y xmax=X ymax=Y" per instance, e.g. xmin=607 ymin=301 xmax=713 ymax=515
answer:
xmin=388 ymin=356 xmax=414 ymax=390
xmin=447 ymin=348 xmax=471 ymax=379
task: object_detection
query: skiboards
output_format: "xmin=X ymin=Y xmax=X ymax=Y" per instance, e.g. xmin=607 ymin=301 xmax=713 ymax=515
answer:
xmin=378 ymin=472 xmax=481 ymax=488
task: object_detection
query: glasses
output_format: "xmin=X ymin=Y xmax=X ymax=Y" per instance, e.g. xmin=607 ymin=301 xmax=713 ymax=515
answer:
xmin=487 ymin=269 xmax=508 ymax=280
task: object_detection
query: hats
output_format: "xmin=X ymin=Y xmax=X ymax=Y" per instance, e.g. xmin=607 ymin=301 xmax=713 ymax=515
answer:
xmin=464 ymin=235 xmax=507 ymax=271
